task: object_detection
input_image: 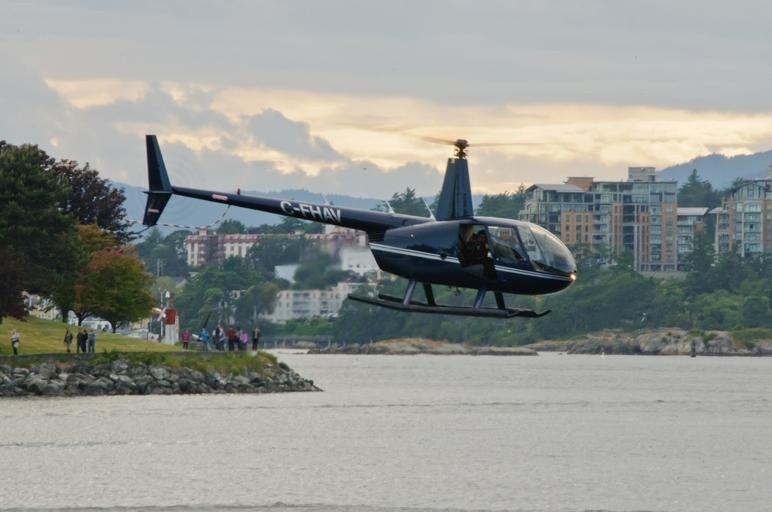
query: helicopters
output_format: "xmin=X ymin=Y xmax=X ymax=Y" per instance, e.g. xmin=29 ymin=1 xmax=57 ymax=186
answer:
xmin=142 ymin=135 xmax=578 ymax=319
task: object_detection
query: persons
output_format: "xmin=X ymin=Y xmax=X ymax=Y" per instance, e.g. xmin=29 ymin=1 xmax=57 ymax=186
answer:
xmin=10 ymin=330 xmax=20 ymax=358
xmin=691 ymin=337 xmax=697 ymax=357
xmin=474 ymin=228 xmax=490 ymax=252
xmin=182 ymin=329 xmax=190 ymax=349
xmin=204 ymin=324 xmax=262 ymax=351
xmin=65 ymin=328 xmax=95 ymax=354
